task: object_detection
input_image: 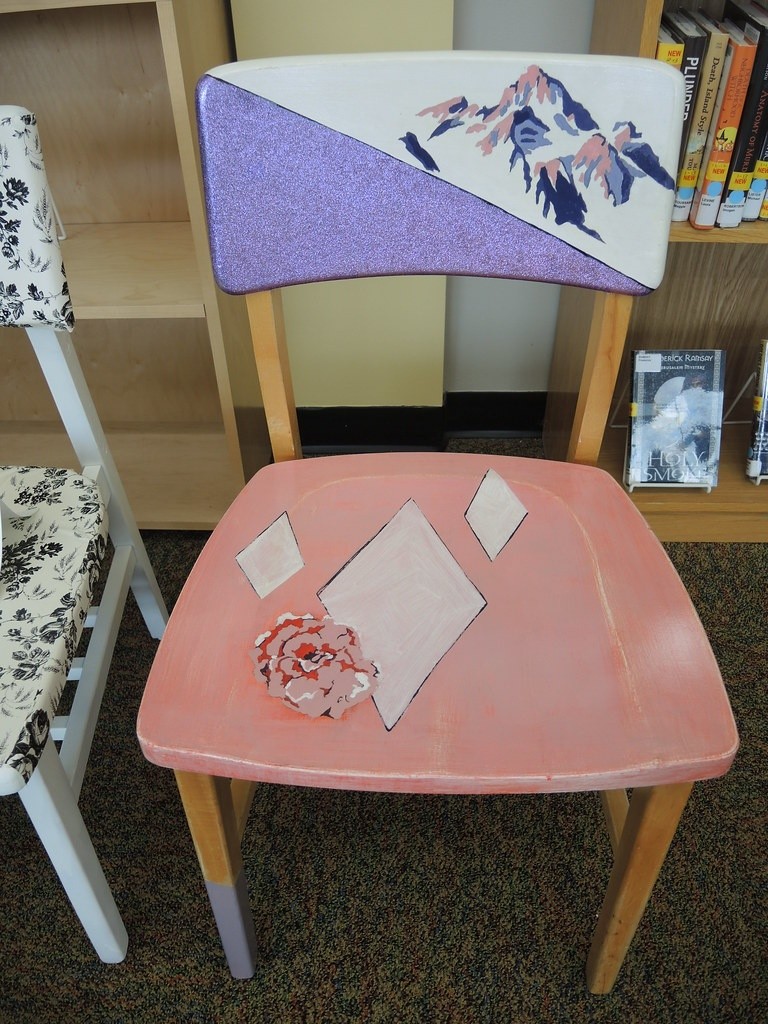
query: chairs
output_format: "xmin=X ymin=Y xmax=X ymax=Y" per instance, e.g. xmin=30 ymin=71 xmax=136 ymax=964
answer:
xmin=1 ymin=103 xmax=169 ymax=963
xmin=137 ymin=50 xmax=739 ymax=997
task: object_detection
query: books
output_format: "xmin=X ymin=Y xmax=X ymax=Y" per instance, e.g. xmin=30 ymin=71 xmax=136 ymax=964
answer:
xmin=624 ymin=348 xmax=727 ymax=487
xmin=655 ymin=0 xmax=768 ymax=231
xmin=743 ymin=338 xmax=768 ymax=478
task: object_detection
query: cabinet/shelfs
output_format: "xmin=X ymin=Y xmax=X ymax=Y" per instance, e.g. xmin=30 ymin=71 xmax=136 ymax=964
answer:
xmin=1 ymin=0 xmax=271 ymax=532
xmin=544 ymin=0 xmax=768 ymax=544
